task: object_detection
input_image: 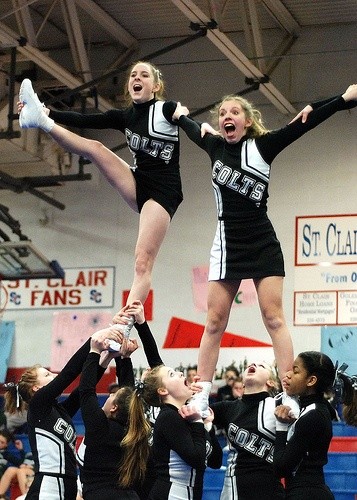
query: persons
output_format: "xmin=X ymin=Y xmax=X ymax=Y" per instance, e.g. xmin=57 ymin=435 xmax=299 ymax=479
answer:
xmin=172 ymin=82 xmax=357 ymax=420
xmin=0 ymin=298 xmax=357 ymax=500
xmin=16 ymin=60 xmax=226 ymax=351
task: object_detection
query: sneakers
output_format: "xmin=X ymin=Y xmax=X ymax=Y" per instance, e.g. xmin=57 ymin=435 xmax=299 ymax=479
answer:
xmin=274 ymin=390 xmax=301 ymax=419
xmin=19 ymin=79 xmax=44 ymax=129
xmin=108 ymin=317 xmax=134 ymax=352
xmin=187 ymin=391 xmax=212 ymax=418
